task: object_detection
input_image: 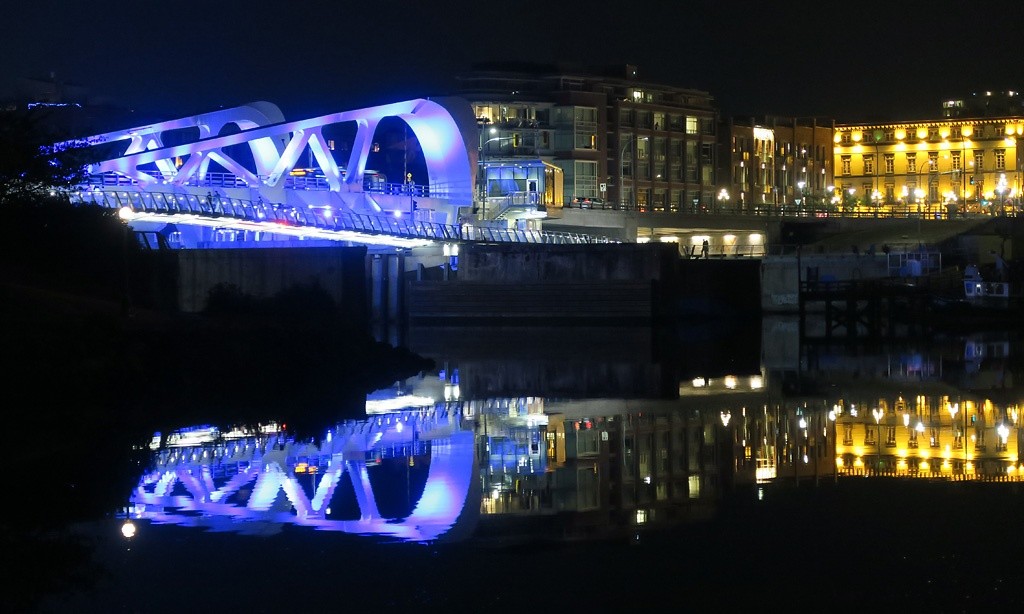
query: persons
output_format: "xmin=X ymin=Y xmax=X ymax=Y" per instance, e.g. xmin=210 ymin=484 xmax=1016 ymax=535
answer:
xmin=701 ymin=239 xmax=710 ymax=257
xmin=206 ymin=191 xmax=212 ymax=204
xmin=257 ymin=196 xmax=263 ymax=206
xmin=213 ymin=191 xmax=221 ymax=201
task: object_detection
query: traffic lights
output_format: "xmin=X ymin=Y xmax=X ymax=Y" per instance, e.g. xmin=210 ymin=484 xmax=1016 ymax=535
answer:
xmin=970 ymin=176 xmax=974 ymax=185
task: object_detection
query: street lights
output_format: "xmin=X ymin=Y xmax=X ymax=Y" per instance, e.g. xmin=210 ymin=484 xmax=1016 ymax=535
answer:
xmin=783 ymin=149 xmax=807 ymax=203
xmin=917 ymin=160 xmax=933 ymax=214
xmin=620 ymin=136 xmax=649 ymax=211
